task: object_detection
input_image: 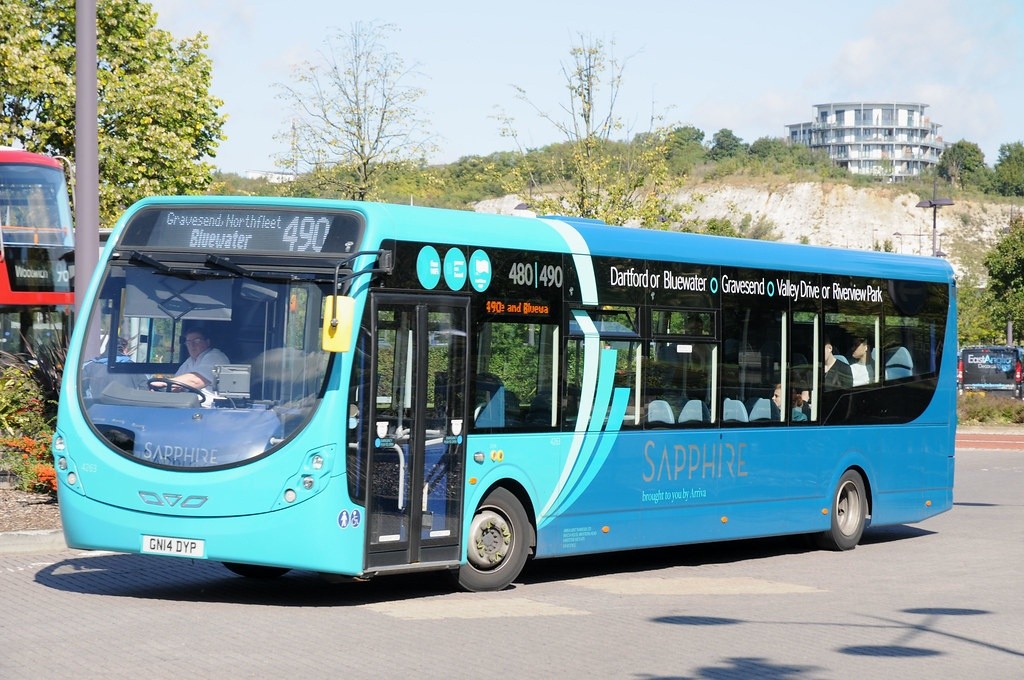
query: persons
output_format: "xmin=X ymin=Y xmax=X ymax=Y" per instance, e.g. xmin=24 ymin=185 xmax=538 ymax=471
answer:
xmin=151 ymin=326 xmax=231 ymax=408
xmin=848 ymin=334 xmax=886 ymax=387
xmin=818 ymin=332 xmax=852 ymax=391
xmin=79 ymin=337 xmax=151 ymax=400
xmin=672 ymin=316 xmax=714 ymax=365
xmin=771 ymin=382 xmax=807 ymax=422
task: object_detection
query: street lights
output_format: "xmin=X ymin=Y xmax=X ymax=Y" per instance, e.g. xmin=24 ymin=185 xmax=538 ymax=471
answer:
xmin=930 ymin=251 xmax=948 ymax=260
xmin=914 ymin=199 xmax=954 ymax=259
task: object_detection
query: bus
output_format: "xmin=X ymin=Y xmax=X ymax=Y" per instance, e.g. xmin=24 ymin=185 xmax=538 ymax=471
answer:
xmin=0 ymin=145 xmax=75 ymax=389
xmin=51 ymin=195 xmax=958 ymax=594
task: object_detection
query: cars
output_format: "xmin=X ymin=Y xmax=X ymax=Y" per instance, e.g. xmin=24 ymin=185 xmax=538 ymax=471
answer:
xmin=957 ymin=345 xmax=1024 ymax=401
xmin=72 ymin=226 xmax=156 ymax=383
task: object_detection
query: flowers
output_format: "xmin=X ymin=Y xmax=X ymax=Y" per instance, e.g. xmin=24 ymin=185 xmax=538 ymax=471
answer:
xmin=32 ymin=464 xmax=55 ymax=484
xmin=50 ymin=479 xmax=57 ymax=490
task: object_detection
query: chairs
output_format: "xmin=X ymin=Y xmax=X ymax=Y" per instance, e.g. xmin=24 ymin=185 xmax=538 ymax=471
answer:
xmin=885 ymin=346 xmax=914 ymax=380
xmin=833 ymin=354 xmax=850 ymax=365
xmin=524 ymin=392 xmax=551 ymax=427
xmin=505 ymin=391 xmax=521 ymax=427
xmin=648 ymin=400 xmax=675 ymax=424
xmin=723 ymin=398 xmax=748 ymax=422
xmin=678 ymin=400 xmax=711 ymax=423
xmin=847 ymin=346 xmax=876 ymax=365
xmin=747 ymin=396 xmax=780 ymax=421
xmin=474 ymin=373 xmax=505 ymax=427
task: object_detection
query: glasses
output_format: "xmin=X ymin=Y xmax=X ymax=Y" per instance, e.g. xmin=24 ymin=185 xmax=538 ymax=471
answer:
xmin=184 ymin=338 xmax=205 ymax=345
